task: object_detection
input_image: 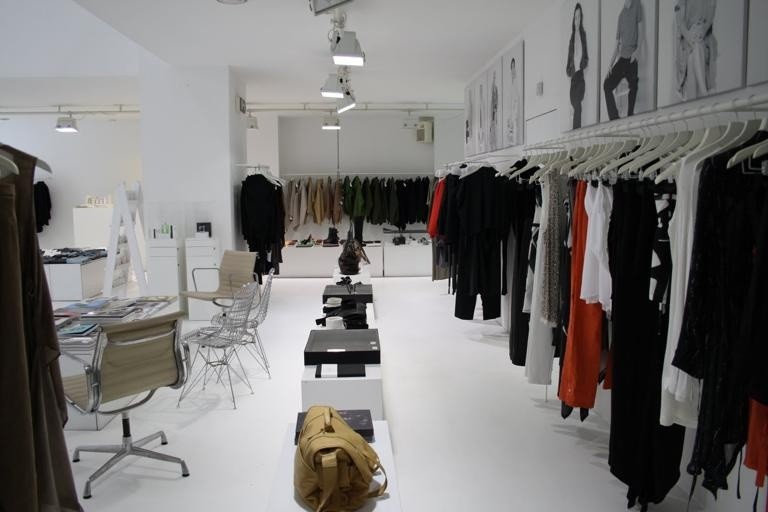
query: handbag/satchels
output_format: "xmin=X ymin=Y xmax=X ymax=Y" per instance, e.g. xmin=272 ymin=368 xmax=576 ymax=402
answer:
xmin=337 ymin=230 xmax=370 ymax=275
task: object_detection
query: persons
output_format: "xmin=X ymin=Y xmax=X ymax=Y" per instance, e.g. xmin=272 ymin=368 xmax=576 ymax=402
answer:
xmin=488 ymin=70 xmax=498 ymax=150
xmin=508 ymin=58 xmax=519 ymax=144
xmin=603 ymin=0 xmax=644 ymax=120
xmin=675 ymin=0 xmax=718 ymax=102
xmin=566 ymin=3 xmax=589 ymax=130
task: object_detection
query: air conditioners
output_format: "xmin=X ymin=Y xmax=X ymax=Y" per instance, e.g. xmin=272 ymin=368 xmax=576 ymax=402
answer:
xmin=416 ymin=121 xmax=432 ymax=144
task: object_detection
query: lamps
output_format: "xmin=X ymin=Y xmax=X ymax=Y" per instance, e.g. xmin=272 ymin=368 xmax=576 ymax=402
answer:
xmin=309 ymin=0 xmax=366 ymax=131
xmin=55 ymin=113 xmax=78 ymax=133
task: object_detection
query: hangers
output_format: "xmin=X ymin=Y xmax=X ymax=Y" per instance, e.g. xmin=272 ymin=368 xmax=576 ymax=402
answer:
xmin=239 ymin=166 xmax=287 ymax=188
xmin=494 ymin=95 xmax=766 ymax=184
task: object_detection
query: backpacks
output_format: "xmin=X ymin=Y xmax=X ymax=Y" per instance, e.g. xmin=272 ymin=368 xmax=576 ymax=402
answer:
xmin=293 ymin=406 xmax=388 ymax=512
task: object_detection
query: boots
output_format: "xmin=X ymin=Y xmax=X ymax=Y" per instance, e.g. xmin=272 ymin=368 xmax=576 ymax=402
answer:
xmin=323 ymin=227 xmax=338 ymax=244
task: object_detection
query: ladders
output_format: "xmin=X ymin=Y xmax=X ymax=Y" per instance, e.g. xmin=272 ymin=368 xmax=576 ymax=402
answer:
xmin=102 ymin=180 xmax=148 ymax=298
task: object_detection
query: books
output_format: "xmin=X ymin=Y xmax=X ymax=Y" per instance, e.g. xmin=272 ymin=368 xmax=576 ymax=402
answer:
xmin=53 ymin=296 xmax=178 ymax=355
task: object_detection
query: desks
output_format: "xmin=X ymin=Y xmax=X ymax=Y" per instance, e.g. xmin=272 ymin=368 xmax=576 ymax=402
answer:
xmin=41 ymin=248 xmax=107 ymax=309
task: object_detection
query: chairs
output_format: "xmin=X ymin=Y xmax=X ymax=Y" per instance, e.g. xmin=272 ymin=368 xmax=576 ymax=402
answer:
xmin=61 ymin=307 xmax=193 ymax=496
xmin=172 ymin=250 xmax=273 ymax=411
